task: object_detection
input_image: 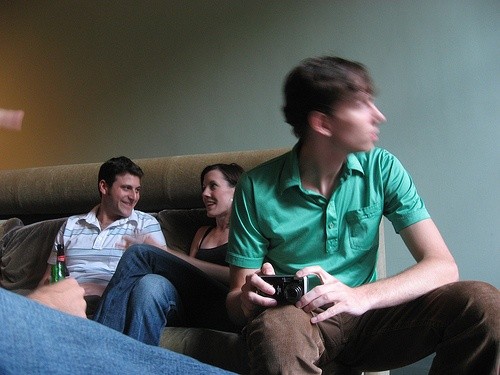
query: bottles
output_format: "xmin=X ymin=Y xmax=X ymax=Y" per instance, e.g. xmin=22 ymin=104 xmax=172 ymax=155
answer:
xmin=50 ymin=244 xmax=69 ymax=284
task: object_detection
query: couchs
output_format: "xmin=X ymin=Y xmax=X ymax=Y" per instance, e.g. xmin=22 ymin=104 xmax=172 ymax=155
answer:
xmin=0 ymin=146 xmax=390 ymax=375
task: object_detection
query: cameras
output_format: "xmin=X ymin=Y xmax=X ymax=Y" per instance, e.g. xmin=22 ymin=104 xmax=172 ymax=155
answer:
xmin=253 ymin=275 xmax=308 ymax=306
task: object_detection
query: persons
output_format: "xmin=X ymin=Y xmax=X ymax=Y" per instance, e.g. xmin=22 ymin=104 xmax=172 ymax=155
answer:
xmin=34 ymin=157 xmax=169 ymax=316
xmin=95 ymin=162 xmax=247 ymax=345
xmin=0 ymin=276 xmax=241 ymax=375
xmin=223 ymin=58 xmax=500 ymax=375
